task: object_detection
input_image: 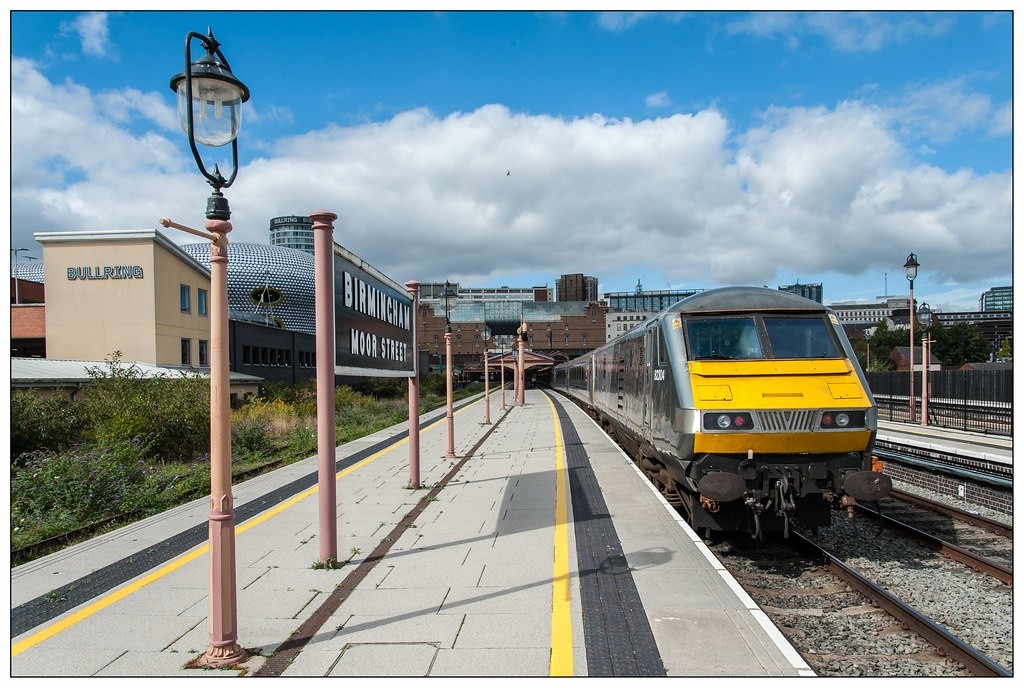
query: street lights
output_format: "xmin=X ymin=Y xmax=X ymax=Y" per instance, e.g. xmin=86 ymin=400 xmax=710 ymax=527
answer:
xmin=917 ymin=301 xmax=933 ymax=426
xmin=13 ymin=247 xmax=29 ymax=304
xmin=903 ymin=253 xmax=920 ymax=423
xmin=480 ymin=321 xmax=492 ymax=423
xmin=169 ymin=25 xmax=252 ymax=668
xmin=23 ymin=256 xmax=37 ymax=280
xmin=512 ymin=346 xmax=518 ymax=402
xmin=438 ymin=280 xmax=458 ymax=459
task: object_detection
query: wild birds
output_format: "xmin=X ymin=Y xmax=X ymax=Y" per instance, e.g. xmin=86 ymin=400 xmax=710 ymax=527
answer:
xmin=507 ymin=170 xmax=511 ymax=176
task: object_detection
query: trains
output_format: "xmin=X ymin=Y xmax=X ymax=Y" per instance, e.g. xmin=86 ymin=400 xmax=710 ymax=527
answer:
xmin=537 ymin=286 xmax=893 ymax=543
